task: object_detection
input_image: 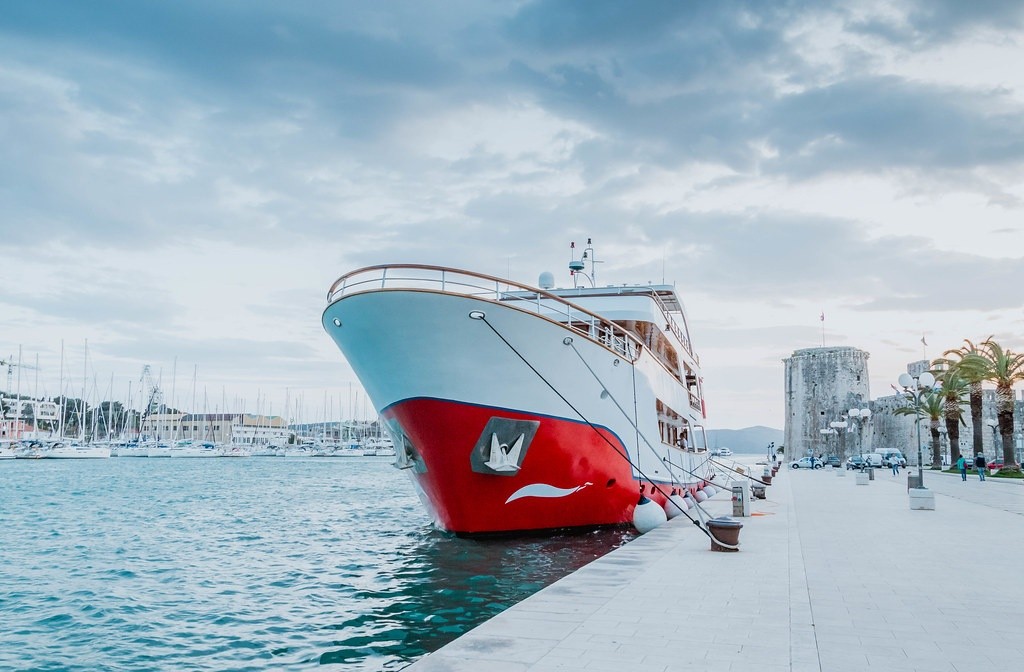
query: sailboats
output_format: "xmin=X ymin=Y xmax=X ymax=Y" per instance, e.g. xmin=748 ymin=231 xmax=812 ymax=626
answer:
xmin=1 ymin=340 xmax=395 ymax=460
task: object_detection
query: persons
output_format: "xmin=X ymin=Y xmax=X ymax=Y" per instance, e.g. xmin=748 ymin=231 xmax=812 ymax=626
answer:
xmin=811 ymin=456 xmax=815 ymax=469
xmin=976 ymin=452 xmax=986 ymax=481
xmin=957 ymin=455 xmax=967 ymax=481
xmin=676 ymin=433 xmax=687 ymax=451
xmin=889 ymin=453 xmax=899 ymax=477
xmin=866 ymin=455 xmax=872 ymax=467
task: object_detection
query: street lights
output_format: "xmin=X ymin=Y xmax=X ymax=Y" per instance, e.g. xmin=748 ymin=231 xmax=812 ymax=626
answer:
xmin=891 ymin=375 xmax=945 ymax=509
xmin=848 ymin=410 xmax=872 ymax=471
xmin=820 ymin=422 xmax=848 ymax=457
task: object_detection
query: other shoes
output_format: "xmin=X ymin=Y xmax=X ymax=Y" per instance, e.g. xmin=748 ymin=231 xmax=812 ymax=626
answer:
xmin=983 ymin=479 xmax=985 ymax=481
xmin=898 ymin=472 xmax=899 ymax=474
xmin=980 ymin=479 xmax=982 ymax=481
xmin=962 ymin=480 xmax=964 ymax=481
xmin=892 ymin=475 xmax=895 ymax=477
xmin=965 ymin=479 xmax=966 ymax=481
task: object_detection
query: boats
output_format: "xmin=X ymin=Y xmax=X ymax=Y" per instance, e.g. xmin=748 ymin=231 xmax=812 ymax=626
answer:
xmin=720 ymin=448 xmax=733 ymax=457
xmin=322 ymin=239 xmax=719 ymax=540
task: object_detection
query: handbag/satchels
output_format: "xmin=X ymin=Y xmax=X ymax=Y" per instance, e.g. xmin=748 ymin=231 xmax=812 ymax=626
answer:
xmin=964 ymin=462 xmax=968 ymax=468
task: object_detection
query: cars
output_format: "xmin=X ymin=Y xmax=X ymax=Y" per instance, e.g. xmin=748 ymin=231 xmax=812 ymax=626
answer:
xmin=829 ymin=456 xmax=841 ymax=467
xmin=789 ymin=457 xmax=823 ymax=469
xmin=846 ymin=456 xmax=868 ymax=471
xmin=937 ymin=426 xmax=948 ymax=467
xmin=987 ymin=458 xmax=1020 ymax=469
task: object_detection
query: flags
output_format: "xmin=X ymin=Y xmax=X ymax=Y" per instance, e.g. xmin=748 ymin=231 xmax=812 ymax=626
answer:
xmin=819 ymin=310 xmax=824 ymax=322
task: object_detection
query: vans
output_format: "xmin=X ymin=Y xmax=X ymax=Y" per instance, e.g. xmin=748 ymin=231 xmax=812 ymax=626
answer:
xmin=874 ymin=448 xmax=906 ymax=468
